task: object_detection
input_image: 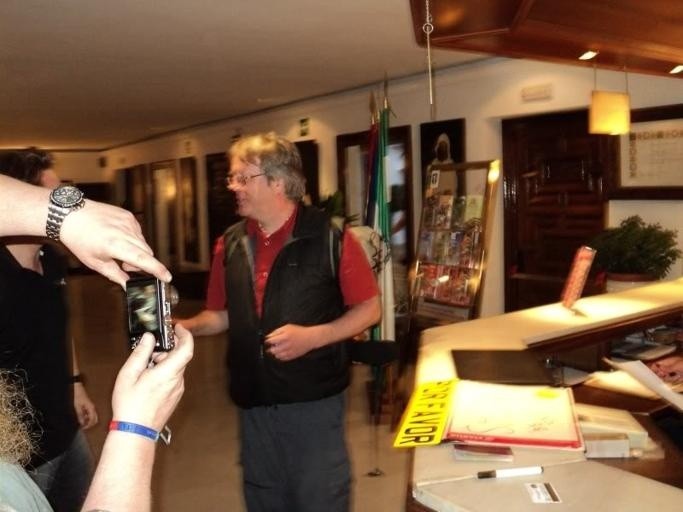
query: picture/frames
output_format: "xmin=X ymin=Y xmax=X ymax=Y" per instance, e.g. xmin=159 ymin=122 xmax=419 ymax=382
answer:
xmin=606 ymin=104 xmax=683 ymax=200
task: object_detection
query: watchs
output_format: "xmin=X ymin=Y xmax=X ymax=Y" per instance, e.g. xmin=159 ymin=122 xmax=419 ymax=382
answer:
xmin=45 ymin=182 xmax=85 ymax=243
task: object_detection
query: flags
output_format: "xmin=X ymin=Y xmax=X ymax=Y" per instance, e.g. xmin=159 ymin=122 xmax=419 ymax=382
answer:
xmin=347 ymin=105 xmax=396 ymax=343
xmin=363 ymin=108 xmax=374 ymax=224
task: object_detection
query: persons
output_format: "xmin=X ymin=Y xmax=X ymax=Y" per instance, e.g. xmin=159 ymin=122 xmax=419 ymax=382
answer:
xmin=0 ymin=168 xmax=194 ymax=511
xmin=165 ymin=131 xmax=385 ymax=511
xmin=424 ymin=133 xmax=461 ymax=201
xmin=1 ymin=144 xmax=99 ymax=511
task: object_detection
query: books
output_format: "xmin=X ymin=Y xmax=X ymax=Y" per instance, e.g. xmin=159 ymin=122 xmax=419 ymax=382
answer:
xmin=409 ymin=192 xmax=485 ymax=308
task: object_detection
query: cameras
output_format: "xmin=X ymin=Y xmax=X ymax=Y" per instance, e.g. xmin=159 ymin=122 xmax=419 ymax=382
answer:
xmin=124 ymin=277 xmax=179 ymax=353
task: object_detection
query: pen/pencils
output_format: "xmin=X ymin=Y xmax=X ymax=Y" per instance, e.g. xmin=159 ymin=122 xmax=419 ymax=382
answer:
xmin=477 ymin=466 xmax=545 ymax=481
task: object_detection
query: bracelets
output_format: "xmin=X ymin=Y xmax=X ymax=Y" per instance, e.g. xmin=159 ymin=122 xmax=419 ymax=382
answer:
xmin=108 ymin=420 xmax=158 ymax=440
xmin=72 ymin=374 xmax=82 ymax=383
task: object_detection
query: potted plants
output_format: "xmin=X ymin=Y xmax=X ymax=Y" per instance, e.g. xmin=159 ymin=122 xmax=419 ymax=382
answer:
xmin=586 ymin=214 xmax=683 ymax=293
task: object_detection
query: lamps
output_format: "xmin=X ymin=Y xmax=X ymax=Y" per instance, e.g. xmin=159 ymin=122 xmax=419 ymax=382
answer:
xmin=586 ymin=61 xmax=632 ymax=136
